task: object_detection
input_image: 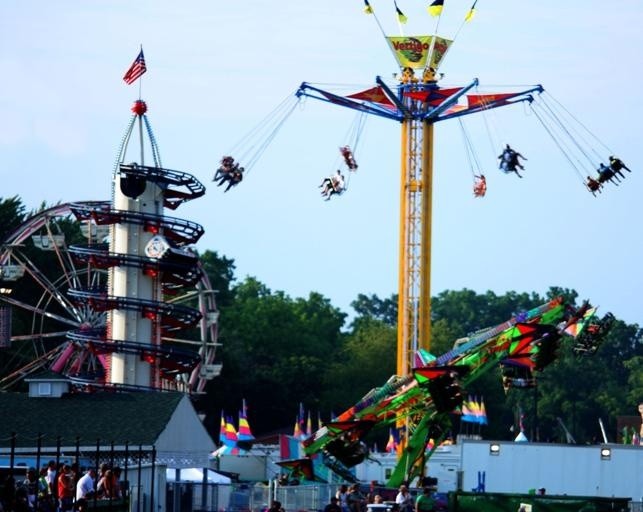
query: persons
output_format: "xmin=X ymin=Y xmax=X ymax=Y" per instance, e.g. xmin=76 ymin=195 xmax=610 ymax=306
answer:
xmin=638 ymin=399 xmax=643 ymax=423
xmin=497 ymin=143 xmax=527 ymax=178
xmin=599 ymin=163 xmax=621 ymax=187
xmin=225 ymin=473 xmax=449 ymax=512
xmin=321 ymin=175 xmax=345 ymax=202
xmin=212 ymin=157 xmax=236 ymax=182
xmin=15 ymin=459 xmax=122 ymax=510
xmin=595 ymin=168 xmax=610 ymax=188
xmin=473 ymin=175 xmax=486 ymax=197
xmin=630 ymin=418 xmax=643 ymax=446
xmin=608 ymin=156 xmax=632 ymax=180
xmin=318 ymin=169 xmax=342 ymax=194
xmin=217 ymin=167 xmax=245 ymax=193
xmin=586 ymin=176 xmax=603 ymax=198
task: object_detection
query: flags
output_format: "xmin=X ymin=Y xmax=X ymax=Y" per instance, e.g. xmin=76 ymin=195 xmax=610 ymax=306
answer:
xmin=426 ymin=0 xmax=444 ymax=16
xmin=364 ymin=0 xmax=374 ymax=15
xmin=464 ymin=1 xmax=478 ymax=24
xmin=393 ymin=1 xmax=408 ymax=24
xmin=122 ymin=45 xmax=146 ymax=86
